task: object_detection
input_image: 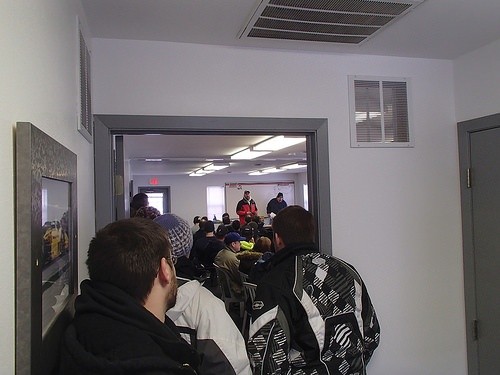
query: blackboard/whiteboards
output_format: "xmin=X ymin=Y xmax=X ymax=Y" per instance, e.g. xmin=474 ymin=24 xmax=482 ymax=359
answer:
xmin=225 ymin=181 xmax=295 ymax=219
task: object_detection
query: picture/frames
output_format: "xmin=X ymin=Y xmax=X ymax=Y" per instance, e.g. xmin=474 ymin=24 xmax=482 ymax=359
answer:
xmin=42 ymin=177 xmax=74 ymax=341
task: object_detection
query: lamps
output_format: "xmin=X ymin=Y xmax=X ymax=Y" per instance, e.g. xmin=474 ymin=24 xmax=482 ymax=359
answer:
xmin=188 ymin=163 xmax=229 ymax=176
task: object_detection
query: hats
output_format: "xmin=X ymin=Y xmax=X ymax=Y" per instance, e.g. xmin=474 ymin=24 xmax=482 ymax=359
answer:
xmin=223 ymin=231 xmax=247 ymax=244
xmin=152 ymin=213 xmax=194 ymax=259
xmin=135 ymin=206 xmax=161 ymax=220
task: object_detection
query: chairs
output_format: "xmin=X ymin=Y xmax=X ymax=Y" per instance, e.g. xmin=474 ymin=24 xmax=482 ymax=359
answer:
xmin=193 ymin=258 xmax=259 ymax=337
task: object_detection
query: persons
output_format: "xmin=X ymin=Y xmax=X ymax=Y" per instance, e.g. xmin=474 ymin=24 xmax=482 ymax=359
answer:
xmin=242 ymin=206 xmax=381 ymax=375
xmin=58 ymin=218 xmax=199 ymax=374
xmin=154 ymin=213 xmax=253 ymax=375
xmin=131 ymin=190 xmax=288 ymax=297
